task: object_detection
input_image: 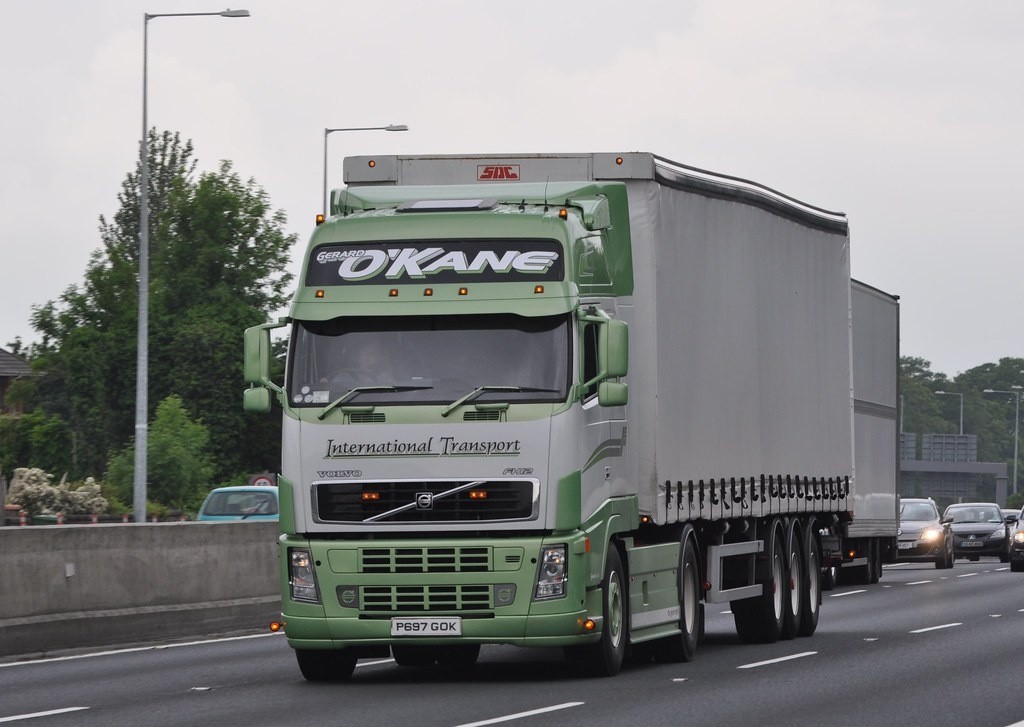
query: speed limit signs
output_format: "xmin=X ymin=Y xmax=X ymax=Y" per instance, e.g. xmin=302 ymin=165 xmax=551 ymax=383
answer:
xmin=253 ymin=477 xmax=273 ymax=486
xmin=249 ymin=475 xmax=276 ymax=486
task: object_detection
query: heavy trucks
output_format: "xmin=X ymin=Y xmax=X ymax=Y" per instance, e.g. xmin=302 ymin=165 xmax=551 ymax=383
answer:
xmin=243 ymin=151 xmax=856 ymax=684
xmin=820 ymin=272 xmax=898 ymax=589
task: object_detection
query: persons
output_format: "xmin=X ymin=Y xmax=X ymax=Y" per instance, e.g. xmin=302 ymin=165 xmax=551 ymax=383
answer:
xmin=321 ymin=343 xmax=391 ymax=383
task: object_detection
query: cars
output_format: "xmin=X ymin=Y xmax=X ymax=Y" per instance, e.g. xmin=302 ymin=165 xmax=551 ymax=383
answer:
xmin=979 ymin=509 xmax=1022 ymax=536
xmin=1005 ymin=505 xmax=1024 ymax=572
xmin=198 ymin=486 xmax=279 ymax=522
xmin=943 ymin=503 xmax=1016 ymax=563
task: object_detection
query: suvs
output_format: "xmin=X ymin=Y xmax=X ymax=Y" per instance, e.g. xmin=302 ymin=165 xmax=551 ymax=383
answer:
xmin=897 ymin=497 xmax=955 ymax=570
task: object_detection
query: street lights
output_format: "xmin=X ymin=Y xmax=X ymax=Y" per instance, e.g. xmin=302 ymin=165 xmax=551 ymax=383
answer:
xmin=323 ymin=124 xmax=409 ymax=221
xmin=134 ymin=8 xmax=251 ymax=522
xmin=935 ymin=391 xmax=963 ymax=434
xmin=984 ymin=389 xmax=1019 ymax=495
